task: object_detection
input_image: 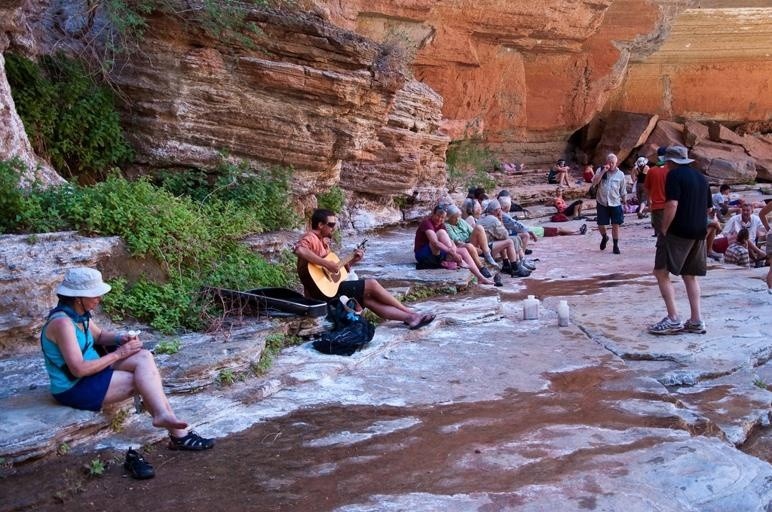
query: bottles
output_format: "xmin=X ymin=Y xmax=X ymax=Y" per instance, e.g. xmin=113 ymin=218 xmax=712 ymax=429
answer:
xmin=128 ymin=331 xmax=136 ymax=341
xmin=558 ymin=300 xmax=569 ymax=326
xmin=522 ymin=294 xmax=539 ymax=320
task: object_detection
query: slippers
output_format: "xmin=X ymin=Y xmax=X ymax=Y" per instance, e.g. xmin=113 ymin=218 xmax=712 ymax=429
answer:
xmin=409 ymin=315 xmax=437 ymax=330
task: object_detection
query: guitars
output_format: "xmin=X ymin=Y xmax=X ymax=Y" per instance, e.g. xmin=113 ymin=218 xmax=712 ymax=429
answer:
xmin=297 ymin=238 xmax=368 ymax=301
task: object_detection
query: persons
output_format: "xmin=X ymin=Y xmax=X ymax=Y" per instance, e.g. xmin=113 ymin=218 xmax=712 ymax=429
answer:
xmin=494 ymin=158 xmax=595 ymax=190
xmin=414 ymin=188 xmax=588 ymax=284
xmin=291 ymin=208 xmax=438 ymax=330
xmin=40 ymin=266 xmax=217 ymax=451
xmin=591 ymin=146 xmax=771 ymax=335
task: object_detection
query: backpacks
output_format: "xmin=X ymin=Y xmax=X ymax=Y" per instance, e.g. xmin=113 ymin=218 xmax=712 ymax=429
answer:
xmin=313 ymin=312 xmax=375 ymax=356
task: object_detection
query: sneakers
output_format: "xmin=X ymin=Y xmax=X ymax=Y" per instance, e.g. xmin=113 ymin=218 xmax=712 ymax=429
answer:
xmin=707 ymin=251 xmax=724 ymax=258
xmin=580 ymin=224 xmax=587 ymax=234
xmin=501 ymin=249 xmax=539 ymax=278
xmin=637 ymin=219 xmax=643 ymax=228
xmin=479 ymin=266 xmax=492 ymax=278
xmin=647 ymin=316 xmax=685 ymax=333
xmin=573 ymin=215 xmax=587 ymax=220
xmin=613 ymin=246 xmax=620 ymax=254
xmin=494 ymin=273 xmax=503 ymax=286
xmin=634 ymin=157 xmax=649 ymax=167
xmin=124 ymin=446 xmax=154 ymax=479
xmin=679 ymin=319 xmax=706 ymax=334
xmin=600 ymin=236 xmax=609 ymax=250
xmin=167 ymin=429 xmax=216 ymax=451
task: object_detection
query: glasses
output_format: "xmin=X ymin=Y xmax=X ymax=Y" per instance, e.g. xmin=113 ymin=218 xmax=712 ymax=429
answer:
xmin=434 ymin=205 xmax=448 ymax=215
xmin=326 ymin=223 xmax=335 ymax=228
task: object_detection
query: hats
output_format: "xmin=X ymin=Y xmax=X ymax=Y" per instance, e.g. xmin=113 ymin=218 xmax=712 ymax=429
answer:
xmin=656 ymin=145 xmax=696 ymax=165
xmin=339 ymin=295 xmax=364 ymax=315
xmin=51 ymin=268 xmax=112 ymax=299
